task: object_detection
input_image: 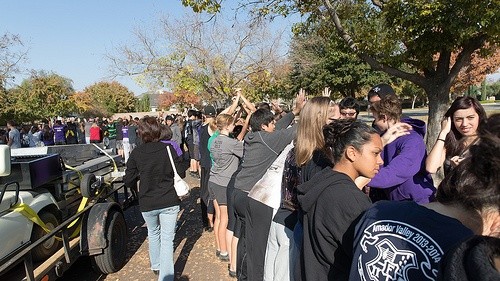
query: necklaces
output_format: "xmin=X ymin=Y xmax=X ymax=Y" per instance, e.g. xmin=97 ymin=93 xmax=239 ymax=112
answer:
xmin=219 ymin=133 xmax=227 ymax=136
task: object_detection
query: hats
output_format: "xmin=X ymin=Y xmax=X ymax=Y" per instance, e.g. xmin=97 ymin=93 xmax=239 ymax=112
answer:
xmin=205 ymin=107 xmax=215 ymax=115
xmin=368 ymin=83 xmax=395 ymax=99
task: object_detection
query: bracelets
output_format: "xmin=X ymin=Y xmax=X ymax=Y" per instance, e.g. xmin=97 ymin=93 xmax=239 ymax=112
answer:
xmin=437 ymin=138 xmax=446 ymax=142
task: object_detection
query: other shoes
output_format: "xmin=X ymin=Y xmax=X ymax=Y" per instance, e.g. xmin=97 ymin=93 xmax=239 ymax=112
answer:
xmin=216 ymin=249 xmax=221 ymax=257
xmin=219 ymin=254 xmax=228 ymax=261
xmin=229 ymin=270 xmax=236 ymax=278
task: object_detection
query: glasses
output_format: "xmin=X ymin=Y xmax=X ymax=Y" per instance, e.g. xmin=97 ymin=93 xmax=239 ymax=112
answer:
xmin=340 ymin=112 xmax=357 ymax=117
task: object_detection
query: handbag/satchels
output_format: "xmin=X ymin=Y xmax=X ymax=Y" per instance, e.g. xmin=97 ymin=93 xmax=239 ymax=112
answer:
xmin=166 ymin=145 xmax=190 ymax=201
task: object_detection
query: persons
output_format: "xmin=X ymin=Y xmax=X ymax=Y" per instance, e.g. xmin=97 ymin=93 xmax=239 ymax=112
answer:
xmin=124 ymin=116 xmax=186 ymax=281
xmin=0 ymin=83 xmax=500 ymax=281
